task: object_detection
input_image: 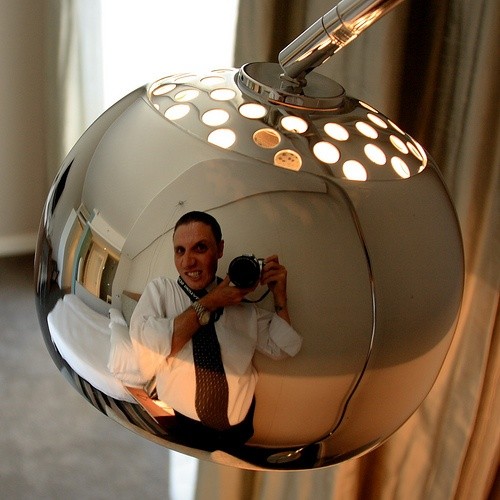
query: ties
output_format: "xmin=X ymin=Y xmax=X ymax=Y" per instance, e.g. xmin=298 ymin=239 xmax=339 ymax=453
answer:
xmin=190 ymin=324 xmax=231 ymax=430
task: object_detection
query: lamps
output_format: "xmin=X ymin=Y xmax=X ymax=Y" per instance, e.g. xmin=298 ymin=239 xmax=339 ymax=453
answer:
xmin=34 ymin=0 xmax=465 ymax=470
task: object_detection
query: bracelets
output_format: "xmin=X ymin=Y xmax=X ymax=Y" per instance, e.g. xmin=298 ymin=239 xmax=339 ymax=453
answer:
xmin=274 ymin=303 xmax=287 ymax=316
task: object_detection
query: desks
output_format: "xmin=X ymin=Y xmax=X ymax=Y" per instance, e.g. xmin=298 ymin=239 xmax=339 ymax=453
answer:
xmin=124 ymin=387 xmax=177 ymax=425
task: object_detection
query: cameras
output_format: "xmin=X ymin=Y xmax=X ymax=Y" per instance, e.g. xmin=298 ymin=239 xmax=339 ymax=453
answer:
xmin=228 ymin=254 xmax=277 ymax=290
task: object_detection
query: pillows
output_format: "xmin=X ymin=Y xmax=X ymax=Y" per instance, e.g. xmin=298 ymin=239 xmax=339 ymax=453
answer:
xmin=107 ymin=323 xmax=147 ymax=386
xmin=109 ymin=306 xmax=129 ymax=328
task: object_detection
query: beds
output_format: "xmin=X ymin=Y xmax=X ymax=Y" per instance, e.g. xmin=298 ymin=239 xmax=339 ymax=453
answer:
xmin=48 ymin=292 xmax=158 ymax=403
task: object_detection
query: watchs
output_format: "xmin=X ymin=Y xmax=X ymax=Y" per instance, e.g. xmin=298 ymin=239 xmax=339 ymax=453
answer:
xmin=191 ymin=301 xmax=213 ymax=326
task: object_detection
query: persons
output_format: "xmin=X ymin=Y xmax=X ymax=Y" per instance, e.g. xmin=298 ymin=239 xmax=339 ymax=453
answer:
xmin=126 ymin=210 xmax=304 ymax=456
